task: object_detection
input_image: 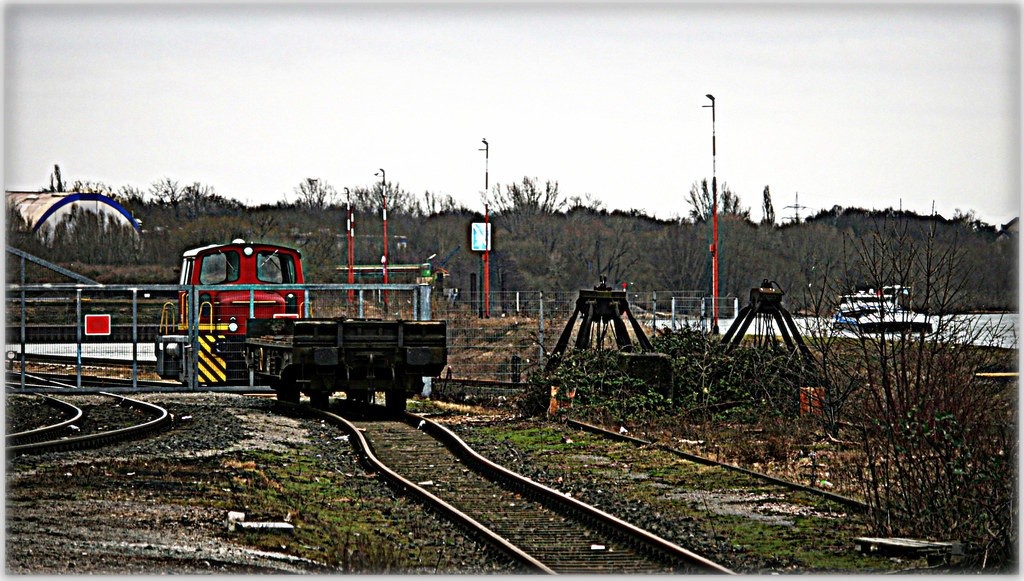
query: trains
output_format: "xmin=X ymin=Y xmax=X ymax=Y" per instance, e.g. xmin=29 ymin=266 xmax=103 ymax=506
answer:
xmin=176 ymin=242 xmax=312 ymax=385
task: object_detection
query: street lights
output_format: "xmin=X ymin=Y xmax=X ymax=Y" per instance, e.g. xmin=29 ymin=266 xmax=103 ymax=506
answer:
xmin=343 ymin=187 xmax=352 ymax=302
xmin=702 ymin=94 xmax=720 ymax=334
xmin=479 ymin=137 xmax=490 ymax=318
xmin=374 ymin=168 xmax=388 ymax=314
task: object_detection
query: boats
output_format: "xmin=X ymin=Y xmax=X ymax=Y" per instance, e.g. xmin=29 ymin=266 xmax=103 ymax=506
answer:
xmin=824 ymin=280 xmax=933 ymax=334
